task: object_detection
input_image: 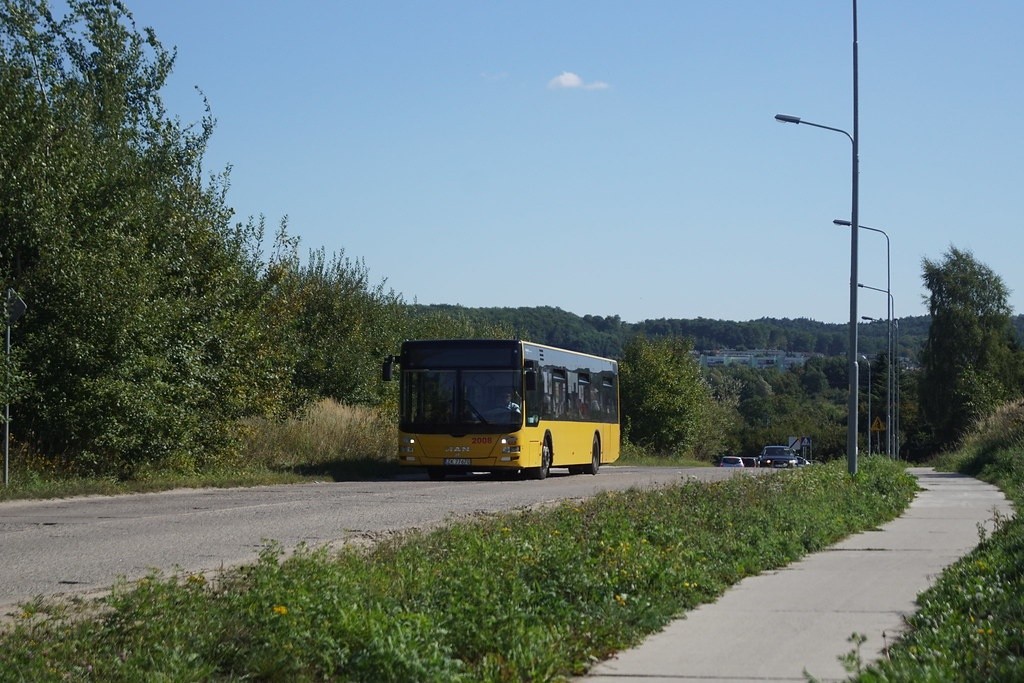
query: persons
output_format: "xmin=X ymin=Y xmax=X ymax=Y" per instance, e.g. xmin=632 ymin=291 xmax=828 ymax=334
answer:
xmin=495 ymin=389 xmax=521 ymax=413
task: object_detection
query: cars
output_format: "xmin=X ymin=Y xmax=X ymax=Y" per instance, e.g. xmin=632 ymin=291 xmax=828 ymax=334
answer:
xmin=720 ymin=456 xmax=745 ymax=468
xmin=797 ymin=456 xmax=809 ymax=467
xmin=759 ymin=445 xmax=799 ymax=468
xmin=742 ymin=457 xmax=761 ymax=468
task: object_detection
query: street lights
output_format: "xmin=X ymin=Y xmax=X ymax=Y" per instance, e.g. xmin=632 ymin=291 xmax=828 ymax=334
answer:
xmin=859 ymin=314 xmax=900 ymax=463
xmin=774 ymin=0 xmax=860 ymax=478
xmin=861 ymin=355 xmax=872 ymax=457
xmin=833 ymin=218 xmax=892 ymax=461
xmin=856 ymin=283 xmax=897 ymax=463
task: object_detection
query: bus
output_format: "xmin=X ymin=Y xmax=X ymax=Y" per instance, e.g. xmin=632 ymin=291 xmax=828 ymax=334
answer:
xmin=382 ymin=339 xmax=621 ymax=481
